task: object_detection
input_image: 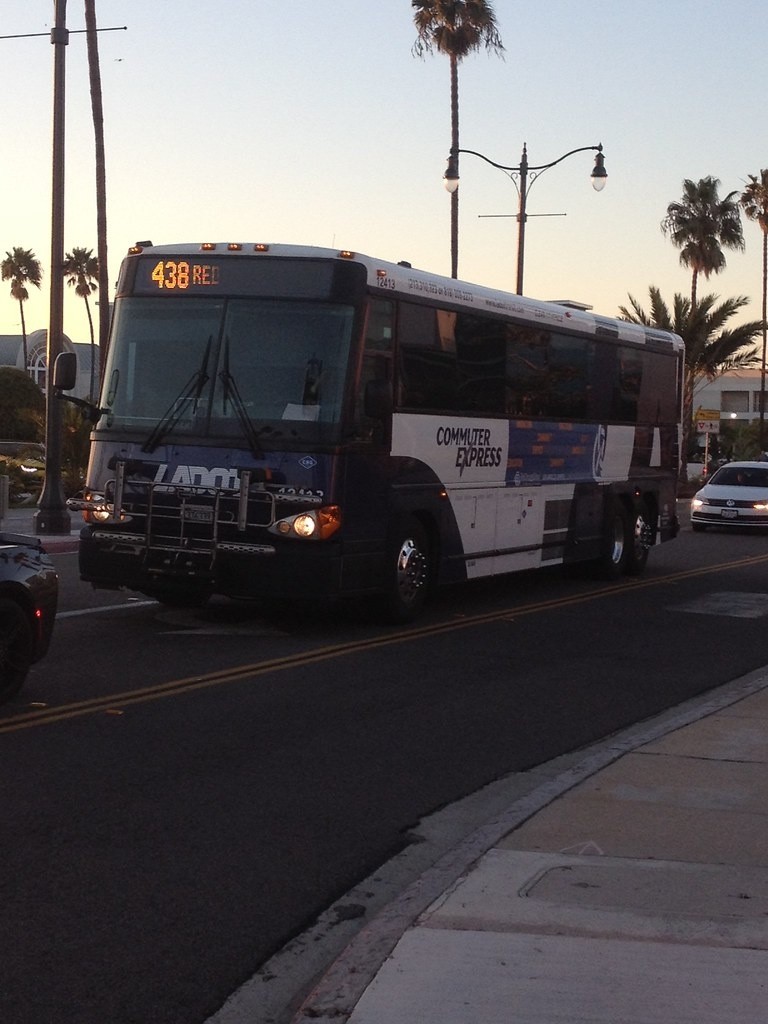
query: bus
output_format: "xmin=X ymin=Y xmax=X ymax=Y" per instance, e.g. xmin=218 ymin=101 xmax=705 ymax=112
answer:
xmin=52 ymin=238 xmax=692 ymax=620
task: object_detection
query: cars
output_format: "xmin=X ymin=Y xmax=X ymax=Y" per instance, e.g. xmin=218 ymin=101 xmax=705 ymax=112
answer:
xmin=0 ymin=528 xmax=60 ymax=711
xmin=689 ymin=461 xmax=768 ymax=533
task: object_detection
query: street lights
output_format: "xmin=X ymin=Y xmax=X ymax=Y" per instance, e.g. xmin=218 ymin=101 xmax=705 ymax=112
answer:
xmin=443 ymin=140 xmax=608 ymax=298
